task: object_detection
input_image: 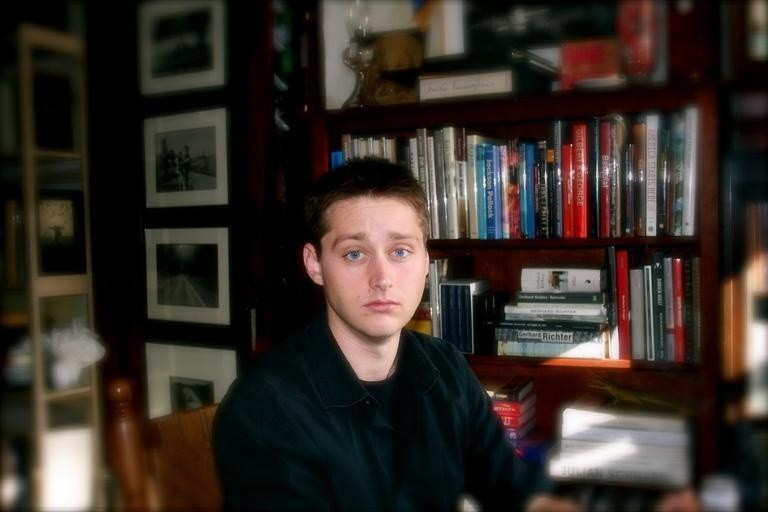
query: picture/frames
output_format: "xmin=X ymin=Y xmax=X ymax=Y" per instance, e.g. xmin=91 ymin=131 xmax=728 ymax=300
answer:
xmin=145 ymin=341 xmax=240 ymax=420
xmin=138 ymin=1 xmax=229 ymax=97
xmin=143 ymin=108 xmax=231 ymax=208
xmin=144 ymin=228 xmax=230 ymax=326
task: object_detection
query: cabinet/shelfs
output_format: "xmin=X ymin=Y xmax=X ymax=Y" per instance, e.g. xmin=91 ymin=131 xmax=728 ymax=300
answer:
xmin=0 ymin=22 xmax=106 ymax=512
xmin=312 ymin=0 xmax=720 ymax=480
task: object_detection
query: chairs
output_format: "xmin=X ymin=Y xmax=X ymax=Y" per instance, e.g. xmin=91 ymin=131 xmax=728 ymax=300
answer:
xmin=108 ymin=379 xmax=225 ymax=512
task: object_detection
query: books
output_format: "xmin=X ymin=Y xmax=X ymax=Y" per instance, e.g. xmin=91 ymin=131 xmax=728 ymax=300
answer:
xmin=479 ymin=375 xmax=693 ymax=488
xmin=418 ymin=51 xmax=513 ymax=105
xmin=404 ymin=245 xmax=700 ymax=363
xmin=333 ymin=108 xmax=700 ymax=239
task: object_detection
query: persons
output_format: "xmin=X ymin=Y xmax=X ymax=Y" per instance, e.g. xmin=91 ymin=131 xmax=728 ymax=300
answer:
xmin=176 ymin=145 xmax=197 ymax=192
xmin=212 ymin=156 xmax=701 ymax=512
